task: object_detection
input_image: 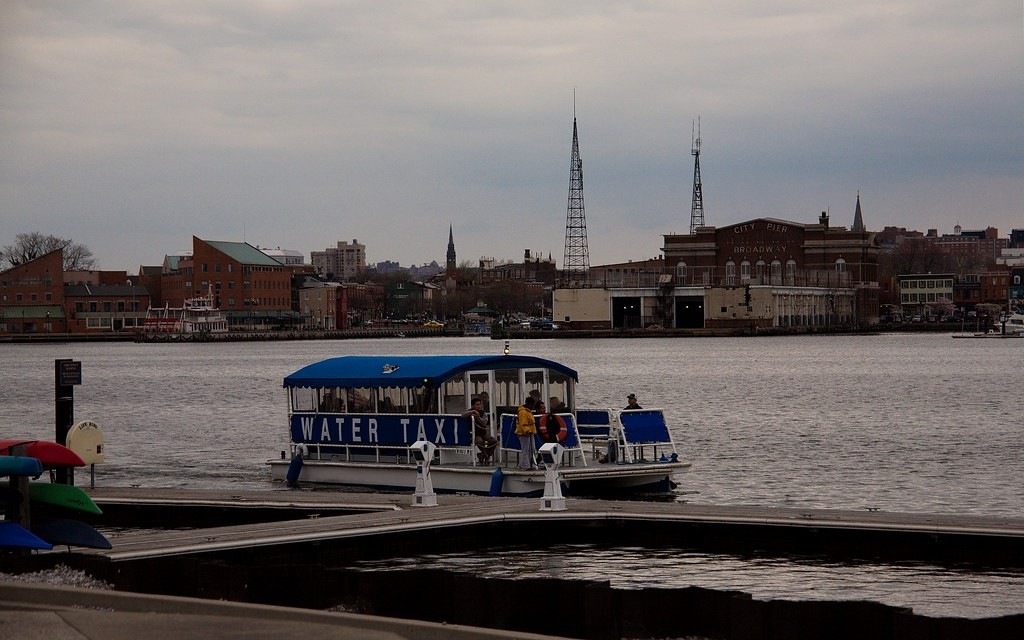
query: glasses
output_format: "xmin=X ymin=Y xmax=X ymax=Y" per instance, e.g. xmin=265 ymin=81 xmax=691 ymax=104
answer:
xmin=476 ymin=404 xmax=481 ymax=406
xmin=481 ymin=396 xmax=488 ymax=398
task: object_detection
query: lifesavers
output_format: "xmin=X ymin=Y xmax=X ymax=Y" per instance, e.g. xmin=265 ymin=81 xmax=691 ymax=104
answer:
xmin=539 ymin=413 xmax=568 ymax=443
xmin=295 ymin=442 xmax=309 ymax=460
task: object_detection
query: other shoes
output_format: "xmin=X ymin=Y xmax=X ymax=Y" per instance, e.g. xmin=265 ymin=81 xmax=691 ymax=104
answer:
xmin=599 ymin=458 xmax=608 ymax=463
xmin=477 ymin=453 xmax=484 ymax=466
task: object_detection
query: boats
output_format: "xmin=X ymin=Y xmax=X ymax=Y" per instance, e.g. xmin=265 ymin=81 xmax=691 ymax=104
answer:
xmin=993 ymin=312 xmax=1024 ymax=335
xmin=143 ymin=280 xmax=230 ymax=333
xmin=264 ymin=354 xmax=693 ymax=501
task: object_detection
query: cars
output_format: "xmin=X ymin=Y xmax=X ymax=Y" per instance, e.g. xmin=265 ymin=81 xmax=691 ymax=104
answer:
xmin=947 ymin=316 xmax=963 ymax=323
xmin=912 ymin=315 xmax=924 ymax=322
xmin=893 ymin=317 xmax=901 ymax=323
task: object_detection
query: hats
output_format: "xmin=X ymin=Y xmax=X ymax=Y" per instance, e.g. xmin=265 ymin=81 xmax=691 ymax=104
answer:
xmin=627 ymin=393 xmax=637 ymax=401
xmin=529 ymin=390 xmax=541 ymax=397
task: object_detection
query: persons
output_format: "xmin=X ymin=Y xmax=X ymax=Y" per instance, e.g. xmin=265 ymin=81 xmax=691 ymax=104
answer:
xmin=483 ymin=392 xmax=489 ymax=413
xmin=525 ymin=389 xmax=543 ymax=413
xmin=462 ymin=398 xmax=500 ymax=465
xmin=534 ymin=401 xmax=545 ymax=463
xmin=599 ymin=393 xmax=643 ymax=464
xmin=551 ymin=398 xmax=567 ymax=412
